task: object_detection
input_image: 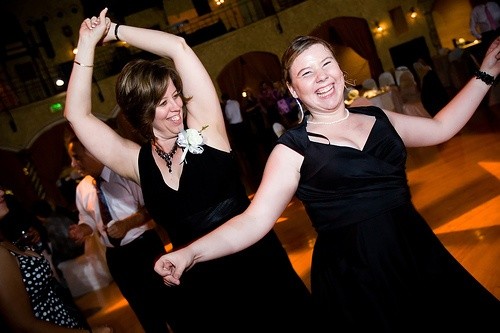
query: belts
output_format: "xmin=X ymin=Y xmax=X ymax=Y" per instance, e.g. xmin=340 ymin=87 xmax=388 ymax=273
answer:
xmin=107 ymin=231 xmax=150 ymax=250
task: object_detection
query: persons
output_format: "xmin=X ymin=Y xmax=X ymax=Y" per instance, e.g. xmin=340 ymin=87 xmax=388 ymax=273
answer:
xmin=221 ymin=93 xmax=244 ymax=135
xmin=20 ymin=206 xmax=78 ymax=284
xmin=469 ymin=0 xmax=500 ymax=56
xmin=153 ymin=36 xmax=500 ymax=333
xmin=0 ymin=188 xmax=90 ymax=333
xmin=254 ymin=78 xmax=287 ymax=125
xmin=68 ymin=137 xmax=167 ymax=333
xmin=62 ymin=7 xmax=312 ymax=333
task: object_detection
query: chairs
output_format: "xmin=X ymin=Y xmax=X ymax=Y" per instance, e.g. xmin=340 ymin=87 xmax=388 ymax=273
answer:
xmin=363 ymin=62 xmax=440 ymax=122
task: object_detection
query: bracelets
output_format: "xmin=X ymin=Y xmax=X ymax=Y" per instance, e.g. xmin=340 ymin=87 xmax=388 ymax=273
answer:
xmin=473 ymin=68 xmax=495 ymax=86
xmin=74 ymin=60 xmax=94 ymax=69
xmin=114 ymin=24 xmax=121 ymax=41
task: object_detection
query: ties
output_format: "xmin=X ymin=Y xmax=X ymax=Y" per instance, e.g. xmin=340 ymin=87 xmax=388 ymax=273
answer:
xmin=94 ymin=177 xmax=121 ymax=249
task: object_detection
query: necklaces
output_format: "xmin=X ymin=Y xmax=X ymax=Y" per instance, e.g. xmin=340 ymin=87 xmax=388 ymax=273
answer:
xmin=307 ymin=107 xmax=349 ymax=125
xmin=154 ymin=137 xmax=180 ymax=173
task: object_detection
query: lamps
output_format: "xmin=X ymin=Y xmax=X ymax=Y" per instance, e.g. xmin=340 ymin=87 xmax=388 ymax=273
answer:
xmin=375 ymin=21 xmax=383 ymax=31
xmin=408 ymin=6 xmax=418 ymax=19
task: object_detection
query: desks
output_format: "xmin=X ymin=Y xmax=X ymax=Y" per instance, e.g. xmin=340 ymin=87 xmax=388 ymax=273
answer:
xmin=344 ymin=85 xmax=402 ymax=115
xmin=58 ymin=235 xmax=112 ymax=297
